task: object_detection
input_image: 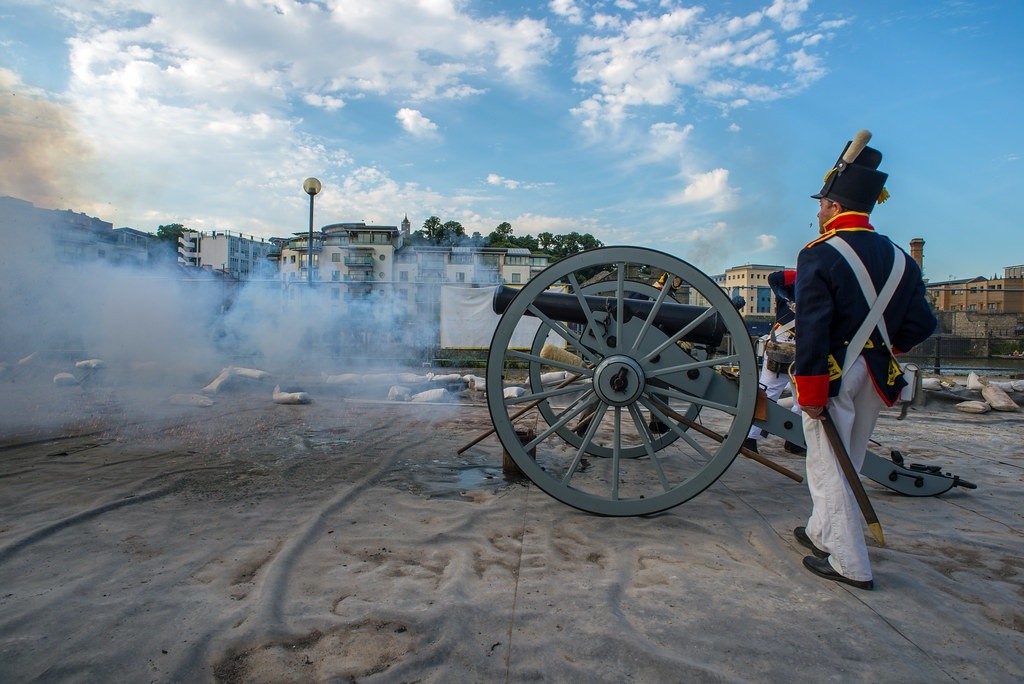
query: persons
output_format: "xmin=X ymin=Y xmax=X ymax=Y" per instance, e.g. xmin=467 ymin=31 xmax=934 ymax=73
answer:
xmin=740 ymin=268 xmax=808 ymax=457
xmin=795 ymin=167 xmax=938 ymax=590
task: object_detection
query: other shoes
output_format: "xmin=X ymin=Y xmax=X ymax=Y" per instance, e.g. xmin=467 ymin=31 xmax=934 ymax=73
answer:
xmin=744 ymin=438 xmax=759 ymax=453
xmin=785 ymin=441 xmax=808 ymax=457
xmin=649 ymin=422 xmax=671 ymax=434
xmin=576 ymin=423 xmax=588 ymax=438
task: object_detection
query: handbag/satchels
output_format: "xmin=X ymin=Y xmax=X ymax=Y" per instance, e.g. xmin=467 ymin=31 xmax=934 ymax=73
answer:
xmin=758 ymin=334 xmax=769 ymax=358
xmin=896 ymin=360 xmax=923 ymax=421
xmin=763 ymin=340 xmax=796 ymax=379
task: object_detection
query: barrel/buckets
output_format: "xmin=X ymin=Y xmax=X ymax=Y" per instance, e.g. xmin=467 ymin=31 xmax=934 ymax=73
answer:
xmin=765 ymin=310 xmax=797 ymax=374
xmin=502 ymin=412 xmax=538 ymax=472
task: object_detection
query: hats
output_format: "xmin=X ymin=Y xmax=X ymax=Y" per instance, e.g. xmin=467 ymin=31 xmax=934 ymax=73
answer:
xmin=810 ymin=130 xmax=890 ymax=214
xmin=656 ymin=272 xmax=683 ymax=297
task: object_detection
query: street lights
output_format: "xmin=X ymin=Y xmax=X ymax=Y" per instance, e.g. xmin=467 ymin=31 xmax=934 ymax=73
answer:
xmin=303 ymin=176 xmax=321 ymax=288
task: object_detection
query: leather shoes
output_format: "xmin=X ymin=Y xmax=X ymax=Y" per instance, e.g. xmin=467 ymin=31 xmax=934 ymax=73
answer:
xmin=802 ymin=555 xmax=873 ymax=590
xmin=794 ymin=527 xmax=831 ymax=559
